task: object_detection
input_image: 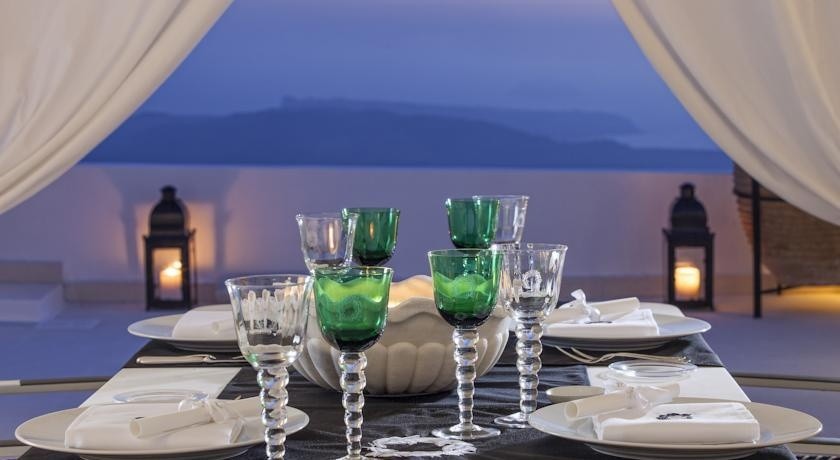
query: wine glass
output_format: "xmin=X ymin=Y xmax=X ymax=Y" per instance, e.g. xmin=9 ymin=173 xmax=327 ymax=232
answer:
xmin=427 ymin=247 xmax=505 ymax=441
xmin=227 ymin=269 xmax=313 ymax=459
xmin=312 ymin=260 xmax=393 ymax=459
xmin=491 ymin=241 xmax=568 ymax=428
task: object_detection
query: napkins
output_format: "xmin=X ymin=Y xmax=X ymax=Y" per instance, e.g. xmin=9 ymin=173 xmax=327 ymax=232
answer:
xmin=591 ymin=402 xmax=763 ymax=448
xmin=68 ymin=402 xmax=245 ymax=453
xmin=173 ymin=309 xmax=240 ymax=340
xmin=541 ymin=306 xmax=662 ymax=339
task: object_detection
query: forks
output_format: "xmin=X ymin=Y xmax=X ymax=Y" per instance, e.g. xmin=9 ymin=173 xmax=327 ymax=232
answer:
xmin=556 ymin=345 xmax=690 ymax=364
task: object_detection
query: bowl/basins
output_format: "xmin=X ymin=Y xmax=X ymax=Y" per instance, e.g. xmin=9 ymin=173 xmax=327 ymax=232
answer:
xmin=172 ymin=304 xmax=238 ymax=341
xmin=286 ymin=268 xmax=516 ymax=395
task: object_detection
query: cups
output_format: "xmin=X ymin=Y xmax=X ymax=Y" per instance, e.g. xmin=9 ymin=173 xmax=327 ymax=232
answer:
xmin=474 ymin=194 xmax=530 ymax=247
xmin=296 ymin=208 xmax=358 ymax=277
xmin=446 ymin=196 xmax=500 ymax=249
xmin=343 ymin=206 xmax=400 ymax=274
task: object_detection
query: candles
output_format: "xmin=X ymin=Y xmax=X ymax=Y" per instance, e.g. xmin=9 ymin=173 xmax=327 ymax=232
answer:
xmin=158 ymin=268 xmax=182 ymax=300
xmin=673 ymin=266 xmax=699 ymax=302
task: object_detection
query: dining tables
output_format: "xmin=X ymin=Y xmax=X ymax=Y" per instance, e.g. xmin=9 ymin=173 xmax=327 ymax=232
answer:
xmin=13 ymin=327 xmax=797 ymax=460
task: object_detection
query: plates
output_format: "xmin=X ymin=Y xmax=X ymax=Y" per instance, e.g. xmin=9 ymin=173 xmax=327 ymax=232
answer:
xmin=129 ymin=310 xmax=245 ymax=354
xmin=510 ymin=309 xmax=712 ymax=351
xmin=546 ymin=386 xmax=605 ymax=403
xmin=607 ymin=357 xmax=697 ymax=383
xmin=527 ymin=393 xmax=823 ymax=460
xmin=112 ymin=387 xmax=208 ymax=403
xmin=15 ymin=399 xmax=310 ymax=458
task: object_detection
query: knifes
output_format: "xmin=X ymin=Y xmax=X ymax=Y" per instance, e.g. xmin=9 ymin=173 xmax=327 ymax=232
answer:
xmin=133 ymin=352 xmax=253 ymax=365
